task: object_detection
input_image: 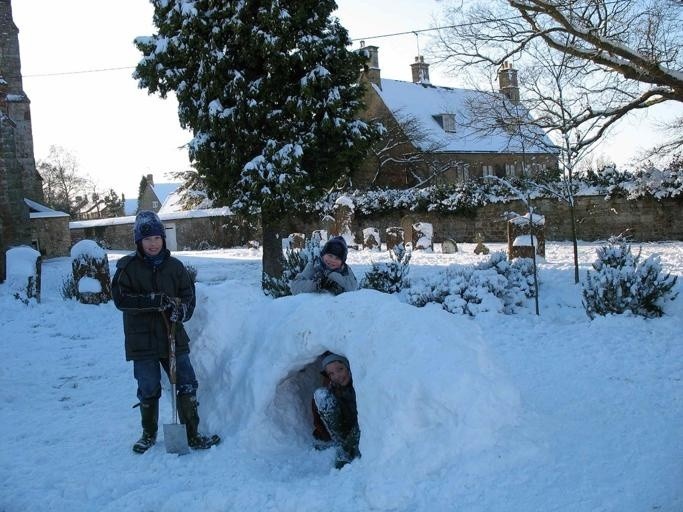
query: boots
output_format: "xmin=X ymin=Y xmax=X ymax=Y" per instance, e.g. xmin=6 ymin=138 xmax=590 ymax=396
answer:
xmin=177 ymin=396 xmax=220 ymax=449
xmin=133 ymin=400 xmax=159 ymax=453
xmin=320 ymin=407 xmax=359 ymax=467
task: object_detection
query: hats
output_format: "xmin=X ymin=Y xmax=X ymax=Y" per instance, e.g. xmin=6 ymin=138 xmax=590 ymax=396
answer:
xmin=321 ymin=236 xmax=348 ymax=262
xmin=321 ymin=351 xmax=349 ymax=368
xmin=134 ymin=210 xmax=163 ymax=241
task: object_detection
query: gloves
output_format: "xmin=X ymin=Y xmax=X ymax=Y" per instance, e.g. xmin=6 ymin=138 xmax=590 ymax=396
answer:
xmin=321 ymin=279 xmax=342 ymax=295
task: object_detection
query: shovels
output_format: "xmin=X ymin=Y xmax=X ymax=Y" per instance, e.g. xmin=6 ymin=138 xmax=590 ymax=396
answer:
xmin=161 ymin=297 xmax=191 ymax=457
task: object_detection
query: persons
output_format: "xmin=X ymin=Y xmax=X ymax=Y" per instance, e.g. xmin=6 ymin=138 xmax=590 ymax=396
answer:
xmin=291 ymin=236 xmax=358 ymax=295
xmin=312 ymin=351 xmax=360 ymax=469
xmin=111 ymin=210 xmax=222 ymax=453
